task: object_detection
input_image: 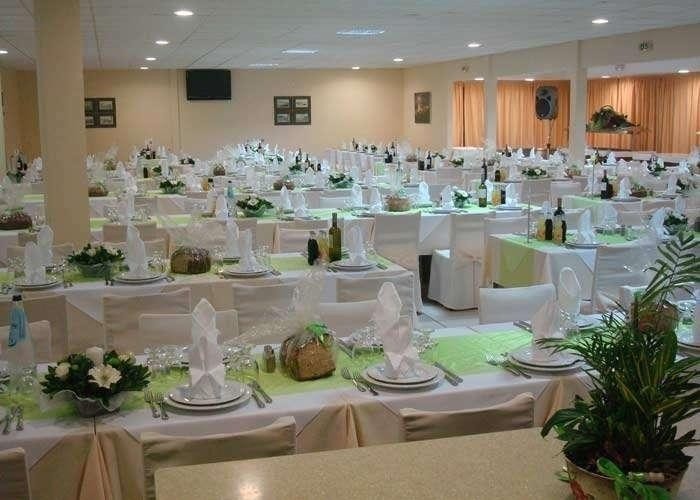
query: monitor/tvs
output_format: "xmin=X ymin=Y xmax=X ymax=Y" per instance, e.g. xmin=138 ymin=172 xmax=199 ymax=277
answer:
xmin=186 ymin=70 xmax=231 ymax=100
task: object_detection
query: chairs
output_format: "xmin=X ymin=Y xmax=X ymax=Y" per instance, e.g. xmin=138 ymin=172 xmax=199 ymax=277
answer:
xmin=1 ymin=144 xmax=698 ymax=500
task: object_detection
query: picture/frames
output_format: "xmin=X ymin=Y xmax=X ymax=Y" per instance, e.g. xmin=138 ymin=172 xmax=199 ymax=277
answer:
xmin=84 ymin=98 xmax=117 ymax=129
xmin=273 ymin=95 xmax=312 ymax=127
xmin=413 ymin=91 xmax=431 ymax=124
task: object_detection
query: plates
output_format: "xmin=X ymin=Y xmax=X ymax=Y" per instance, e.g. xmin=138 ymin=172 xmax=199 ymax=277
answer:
xmin=162 ymin=383 xmax=253 ymax=409
xmin=677 ymin=335 xmax=699 ymax=351
xmin=361 ymin=364 xmax=445 ymax=389
xmin=509 ymin=343 xmax=585 ymax=371
xmin=578 ymin=314 xmax=600 ymax=330
xmin=347 ymin=200 xmax=465 ymax=218
xmin=10 ymin=245 xmax=376 ymax=291
xmin=566 ymin=241 xmax=604 ymax=248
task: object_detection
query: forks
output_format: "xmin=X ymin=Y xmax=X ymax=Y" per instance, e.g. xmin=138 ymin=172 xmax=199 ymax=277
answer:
xmin=354 ymin=371 xmax=379 ymax=394
xmin=341 ymin=367 xmax=365 ymax=392
xmin=142 ymin=388 xmax=169 ymax=421
xmin=484 ymin=352 xmax=532 ymax=380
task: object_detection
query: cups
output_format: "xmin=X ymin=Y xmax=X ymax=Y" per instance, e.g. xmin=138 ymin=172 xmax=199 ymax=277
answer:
xmin=352 ymin=319 xmax=439 ymax=366
xmin=0 ymin=352 xmax=39 ymax=410
xmin=676 ymin=300 xmax=696 ymax=335
xmin=592 ymin=204 xmax=641 ymax=241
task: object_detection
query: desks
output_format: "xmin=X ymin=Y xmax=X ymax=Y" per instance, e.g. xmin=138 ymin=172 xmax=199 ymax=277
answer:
xmin=565 ymin=123 xmax=643 ymax=151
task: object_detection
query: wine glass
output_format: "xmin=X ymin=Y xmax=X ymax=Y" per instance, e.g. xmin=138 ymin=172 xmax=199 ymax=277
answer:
xmin=146 ymin=339 xmax=260 ymax=396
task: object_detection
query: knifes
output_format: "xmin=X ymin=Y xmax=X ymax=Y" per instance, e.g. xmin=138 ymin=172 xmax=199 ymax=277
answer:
xmin=248 ymin=381 xmax=272 ymax=408
xmin=433 ymin=360 xmax=463 ymax=387
xmin=4 ymin=404 xmax=23 ymax=434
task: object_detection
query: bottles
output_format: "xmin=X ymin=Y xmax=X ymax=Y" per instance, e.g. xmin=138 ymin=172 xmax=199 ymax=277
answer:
xmin=552 ymin=215 xmax=563 ymax=245
xmin=600 ymin=170 xmax=609 ymax=199
xmin=7 ymin=294 xmax=36 ymax=397
xmin=481 ymin=158 xmax=487 ymax=183
xmin=608 ymin=178 xmax=617 ymax=196
xmin=492 ymin=182 xmax=507 ymax=203
xmin=535 ymin=213 xmax=546 ymax=240
xmin=495 ymin=163 xmax=505 ymax=181
xmin=545 ymin=212 xmax=552 ymax=241
xmin=478 ymin=178 xmax=487 ymax=207
xmin=343 ymin=137 xmax=463 ymax=172
xmin=205 ymin=176 xmax=238 ymax=220
xmin=329 ymin=213 xmax=341 ymax=262
xmin=307 ymin=230 xmax=329 ymax=263
xmin=296 ymin=148 xmax=322 ymax=175
xmin=262 ymin=346 xmax=276 ymax=374
xmin=552 ymin=198 xmax=566 ymax=241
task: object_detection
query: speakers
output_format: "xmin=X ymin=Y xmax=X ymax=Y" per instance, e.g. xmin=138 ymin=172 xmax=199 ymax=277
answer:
xmin=535 ymin=86 xmax=559 ymax=120
